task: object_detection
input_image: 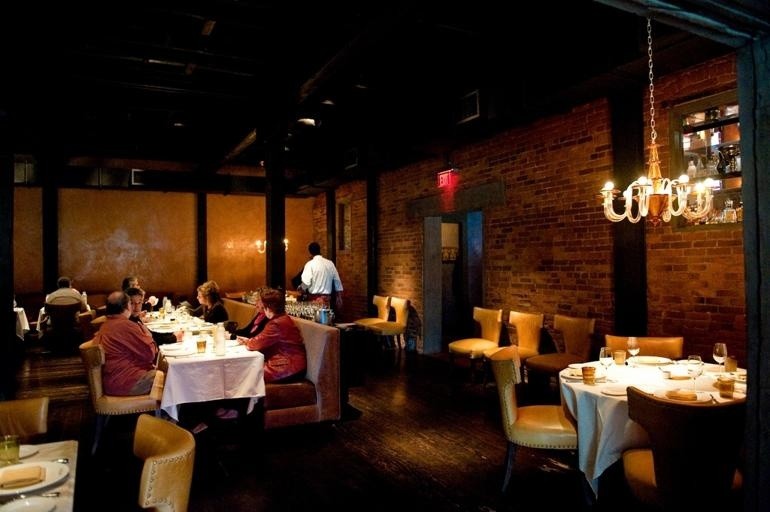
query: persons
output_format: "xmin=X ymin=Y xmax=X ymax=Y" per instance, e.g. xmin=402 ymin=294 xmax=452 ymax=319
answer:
xmin=300 ymin=243 xmax=344 ymax=312
xmin=46 ymin=276 xmax=307 ymax=419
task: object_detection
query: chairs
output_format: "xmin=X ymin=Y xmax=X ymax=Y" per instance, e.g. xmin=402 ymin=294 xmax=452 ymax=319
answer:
xmin=623 ymin=387 xmax=747 ymax=511
xmin=483 ymin=310 xmax=545 ymax=383
xmin=605 ymin=334 xmax=684 ymax=359
xmin=525 ymin=314 xmax=597 ymax=390
xmin=353 ymin=295 xmax=391 ymax=348
xmin=448 ymin=307 xmax=504 ymax=397
xmin=369 ymin=297 xmax=411 ymax=350
xmin=133 ymin=414 xmax=195 ymax=512
xmin=489 ymin=345 xmax=578 ymax=492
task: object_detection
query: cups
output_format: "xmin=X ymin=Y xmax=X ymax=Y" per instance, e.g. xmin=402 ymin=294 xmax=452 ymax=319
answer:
xmin=615 ymin=350 xmax=626 ymax=364
xmin=315 ymin=308 xmax=334 ymax=325
xmin=0 ymin=435 xmax=19 ymax=464
xmin=159 ymin=306 xmax=226 ymax=356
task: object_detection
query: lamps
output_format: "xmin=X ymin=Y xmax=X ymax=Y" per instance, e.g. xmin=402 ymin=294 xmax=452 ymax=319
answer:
xmin=600 ymin=20 xmax=716 ymax=229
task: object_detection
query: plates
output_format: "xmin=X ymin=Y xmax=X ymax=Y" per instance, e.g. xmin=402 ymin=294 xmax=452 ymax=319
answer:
xmin=142 ymin=311 xmax=173 ymax=334
xmin=227 ymin=340 xmax=240 ymax=346
xmin=18 ymin=444 xmax=40 ymax=460
xmin=0 ymin=459 xmax=69 ymax=509
xmin=564 ymin=356 xmax=747 ymax=401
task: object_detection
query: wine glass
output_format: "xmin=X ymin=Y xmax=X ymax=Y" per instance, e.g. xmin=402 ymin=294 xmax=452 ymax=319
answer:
xmin=713 ymin=344 xmax=728 ymax=373
xmin=626 ymin=337 xmax=640 ymax=366
xmin=287 ymin=301 xmax=330 ymax=319
xmin=600 ymin=346 xmax=615 ymax=376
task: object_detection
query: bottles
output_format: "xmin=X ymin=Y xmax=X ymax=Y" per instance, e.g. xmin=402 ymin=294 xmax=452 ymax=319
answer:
xmin=82 ymin=291 xmax=88 ymax=305
xmin=441 ymin=247 xmax=457 ymax=262
xmin=686 ymin=155 xmax=724 ymax=176
xmin=163 ymin=296 xmax=176 ymax=320
xmin=683 ymin=199 xmax=743 ymax=225
